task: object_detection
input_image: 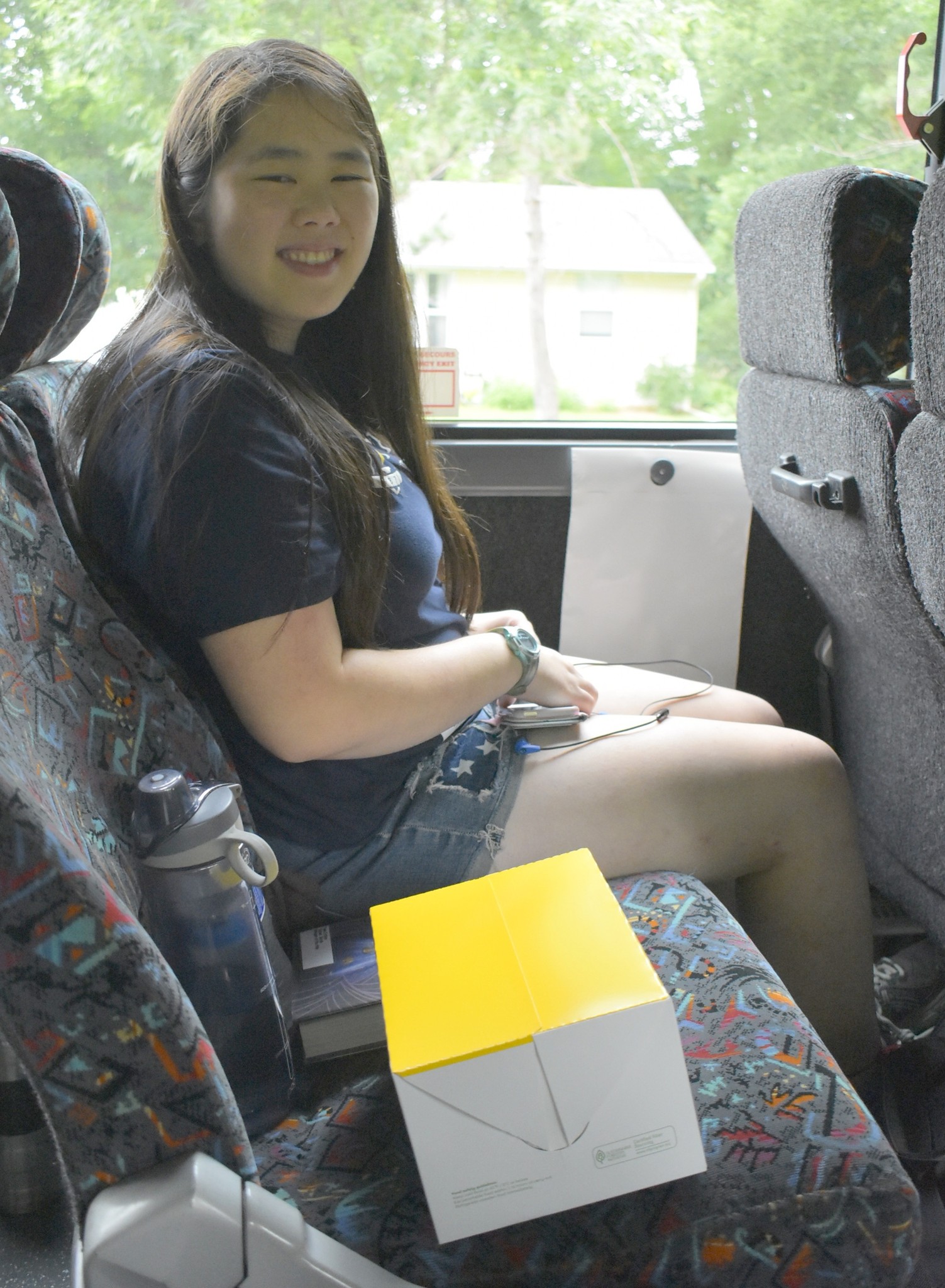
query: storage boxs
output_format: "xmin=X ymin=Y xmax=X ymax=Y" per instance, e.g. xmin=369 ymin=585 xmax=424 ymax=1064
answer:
xmin=368 ymin=850 xmax=704 ymax=1250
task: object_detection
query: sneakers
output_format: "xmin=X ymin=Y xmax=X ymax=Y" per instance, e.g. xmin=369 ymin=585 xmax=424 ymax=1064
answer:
xmin=871 ymin=934 xmax=945 ymax=1023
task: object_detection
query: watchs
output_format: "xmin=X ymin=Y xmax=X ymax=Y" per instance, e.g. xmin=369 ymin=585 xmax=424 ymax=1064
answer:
xmin=487 ymin=626 xmax=541 ymax=696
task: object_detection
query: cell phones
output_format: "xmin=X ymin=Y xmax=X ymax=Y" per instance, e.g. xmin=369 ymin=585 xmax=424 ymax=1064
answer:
xmin=507 ymin=703 xmax=579 ymax=719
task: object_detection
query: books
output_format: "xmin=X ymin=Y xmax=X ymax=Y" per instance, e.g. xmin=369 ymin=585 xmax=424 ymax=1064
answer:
xmin=292 ymin=933 xmax=388 ymax=1066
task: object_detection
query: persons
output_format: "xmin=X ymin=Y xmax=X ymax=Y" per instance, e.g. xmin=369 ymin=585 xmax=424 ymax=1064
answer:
xmin=56 ymin=39 xmax=945 ymax=1190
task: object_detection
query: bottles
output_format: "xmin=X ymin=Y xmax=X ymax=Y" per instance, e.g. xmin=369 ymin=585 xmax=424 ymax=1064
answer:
xmin=129 ymin=767 xmax=300 ymax=1136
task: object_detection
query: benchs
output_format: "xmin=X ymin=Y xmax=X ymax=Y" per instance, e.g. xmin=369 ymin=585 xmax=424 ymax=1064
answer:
xmin=737 ymin=171 xmax=944 ymax=939
xmin=3 ymin=142 xmax=920 ymax=1288
